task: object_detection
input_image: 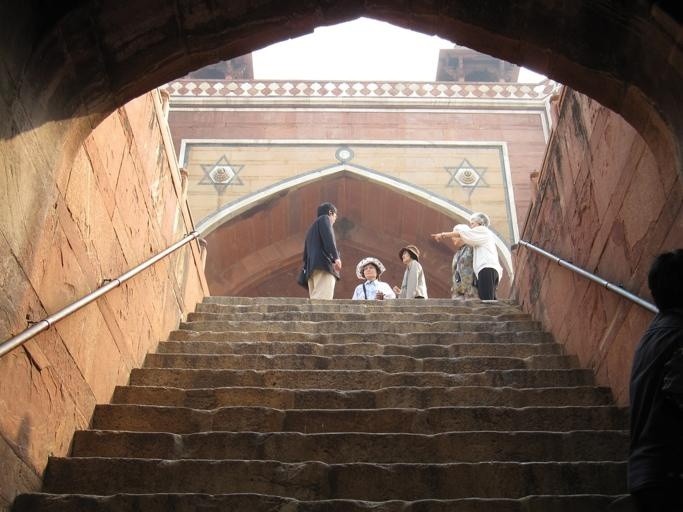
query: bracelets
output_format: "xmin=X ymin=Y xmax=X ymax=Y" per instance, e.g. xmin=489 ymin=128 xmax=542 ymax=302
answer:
xmin=441 ymin=232 xmax=446 ymax=239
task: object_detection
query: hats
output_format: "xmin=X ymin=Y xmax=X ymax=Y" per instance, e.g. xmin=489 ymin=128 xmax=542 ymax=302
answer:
xmin=399 ymin=245 xmax=419 ymax=261
xmin=356 ymin=257 xmax=385 ymax=280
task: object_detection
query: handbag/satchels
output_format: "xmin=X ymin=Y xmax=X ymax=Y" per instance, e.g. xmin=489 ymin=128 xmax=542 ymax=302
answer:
xmin=298 ymin=269 xmax=308 ymax=288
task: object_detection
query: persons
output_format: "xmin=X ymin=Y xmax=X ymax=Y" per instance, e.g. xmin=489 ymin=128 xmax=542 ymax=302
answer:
xmin=429 ymin=213 xmax=513 ymax=299
xmin=351 ymin=257 xmax=396 ymax=300
xmin=628 ymin=247 xmax=683 ymax=511
xmin=304 ymin=201 xmax=342 ymax=299
xmin=450 ymin=223 xmax=480 ymax=299
xmin=392 ymin=244 xmax=428 ymax=298
